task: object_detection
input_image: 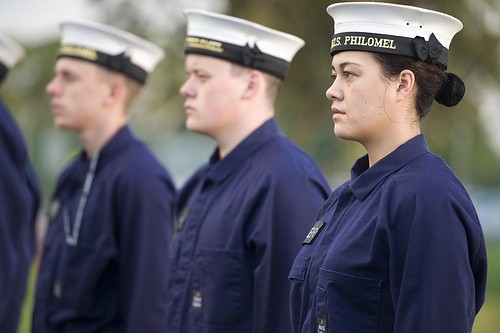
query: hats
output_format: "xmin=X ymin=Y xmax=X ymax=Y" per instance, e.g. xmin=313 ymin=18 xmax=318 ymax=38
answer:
xmin=326 ymin=1 xmax=463 ymax=68
xmin=0 ymin=32 xmax=26 ymax=70
xmin=183 ymin=9 xmax=305 ymax=79
xmin=55 ymin=17 xmax=165 ymax=84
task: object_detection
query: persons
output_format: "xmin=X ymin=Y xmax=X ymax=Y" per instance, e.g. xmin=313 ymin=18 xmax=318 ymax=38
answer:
xmin=31 ymin=19 xmax=178 ymax=333
xmin=0 ymin=32 xmax=42 ymax=333
xmin=168 ymin=9 xmax=332 ymax=333
xmin=289 ymin=2 xmax=488 ymax=333
